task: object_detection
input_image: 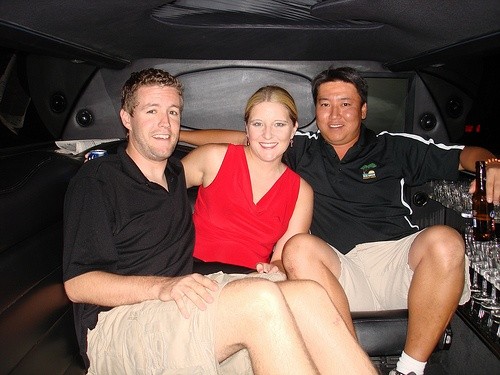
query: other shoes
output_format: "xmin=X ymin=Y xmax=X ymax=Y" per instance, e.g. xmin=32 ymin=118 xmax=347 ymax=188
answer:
xmin=388 ymin=367 xmax=416 ymax=375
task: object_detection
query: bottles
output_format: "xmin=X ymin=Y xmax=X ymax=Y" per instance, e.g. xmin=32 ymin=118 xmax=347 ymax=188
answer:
xmin=473 ymin=161 xmax=494 ymax=242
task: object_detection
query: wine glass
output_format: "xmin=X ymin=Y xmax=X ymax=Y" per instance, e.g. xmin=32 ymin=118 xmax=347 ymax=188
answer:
xmin=464 ymin=225 xmax=500 ymax=325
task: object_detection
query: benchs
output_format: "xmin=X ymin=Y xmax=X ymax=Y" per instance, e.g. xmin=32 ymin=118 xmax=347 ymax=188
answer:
xmin=0 ymin=57 xmax=454 ymax=375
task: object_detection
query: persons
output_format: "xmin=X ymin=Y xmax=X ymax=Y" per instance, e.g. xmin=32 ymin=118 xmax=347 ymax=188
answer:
xmin=179 ymin=86 xmax=314 ymax=283
xmin=62 ymin=68 xmax=378 ymax=375
xmin=177 ymin=64 xmax=500 ymax=375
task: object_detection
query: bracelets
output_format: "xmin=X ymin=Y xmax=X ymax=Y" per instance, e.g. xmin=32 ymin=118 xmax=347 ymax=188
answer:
xmin=484 ymin=158 xmax=500 ymax=165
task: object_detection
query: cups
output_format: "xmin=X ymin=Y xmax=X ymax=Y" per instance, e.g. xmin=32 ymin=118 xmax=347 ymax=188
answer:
xmin=431 ymin=179 xmax=499 ymax=219
